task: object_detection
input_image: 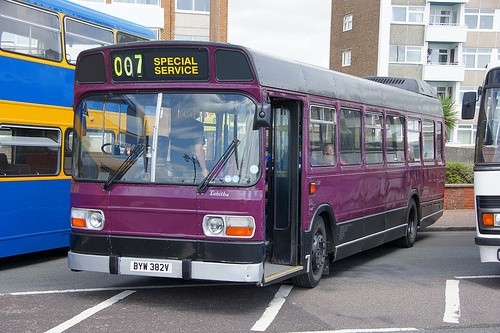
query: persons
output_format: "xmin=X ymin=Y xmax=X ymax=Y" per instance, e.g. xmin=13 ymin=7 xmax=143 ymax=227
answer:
xmin=322 ymin=144 xmax=344 ymax=165
xmin=0 ymin=153 xmax=20 ymax=175
xmin=169 ymin=106 xmax=208 ymax=178
xmin=27 ymin=147 xmax=57 ymax=174
xmin=113 ymin=144 xmax=147 ymax=155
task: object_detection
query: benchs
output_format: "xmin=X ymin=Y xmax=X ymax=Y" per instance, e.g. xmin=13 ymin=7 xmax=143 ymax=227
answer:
xmin=341 ymin=147 xmax=404 ymax=163
xmin=0 ymin=151 xmax=58 ymax=175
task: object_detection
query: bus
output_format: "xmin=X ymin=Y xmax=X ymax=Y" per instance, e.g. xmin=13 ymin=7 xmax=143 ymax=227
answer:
xmin=69 ymin=39 xmax=444 ymax=290
xmin=0 ymin=0 xmax=157 ymax=259
xmin=461 ymin=61 xmax=500 ymax=264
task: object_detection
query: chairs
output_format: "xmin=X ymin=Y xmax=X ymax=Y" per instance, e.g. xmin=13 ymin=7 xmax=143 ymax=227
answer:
xmin=1 ymin=41 xmax=70 ymax=63
